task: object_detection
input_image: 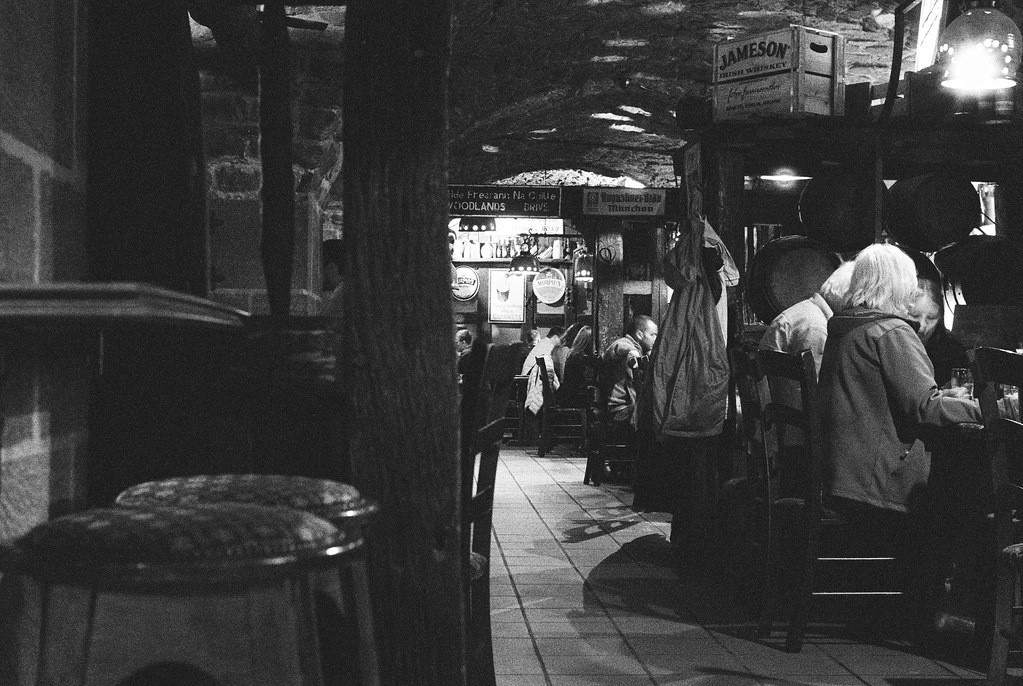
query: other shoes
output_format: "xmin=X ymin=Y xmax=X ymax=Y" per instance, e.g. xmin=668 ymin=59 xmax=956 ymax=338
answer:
xmin=934 ymin=608 xmax=975 ymax=638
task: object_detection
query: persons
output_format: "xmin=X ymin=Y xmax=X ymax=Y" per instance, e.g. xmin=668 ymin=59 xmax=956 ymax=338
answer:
xmin=520 ymin=315 xmax=659 ymax=424
xmin=757 ymin=243 xmax=1019 ymax=646
xmin=453 ymin=328 xmax=471 ymax=375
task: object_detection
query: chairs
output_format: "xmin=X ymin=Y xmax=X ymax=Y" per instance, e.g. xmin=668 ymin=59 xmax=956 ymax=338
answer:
xmin=455 ymin=335 xmax=1023 ymax=686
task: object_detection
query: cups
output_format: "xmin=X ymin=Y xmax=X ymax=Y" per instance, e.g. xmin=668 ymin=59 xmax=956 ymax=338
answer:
xmin=1003 ymin=385 xmax=1019 ymax=421
xmin=951 ymin=368 xmax=974 ymax=401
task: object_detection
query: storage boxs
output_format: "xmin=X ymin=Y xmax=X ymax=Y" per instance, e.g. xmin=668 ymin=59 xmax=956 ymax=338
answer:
xmin=708 ymin=27 xmax=846 ymax=117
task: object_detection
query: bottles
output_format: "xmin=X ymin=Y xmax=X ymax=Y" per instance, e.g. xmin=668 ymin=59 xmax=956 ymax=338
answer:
xmin=453 ymin=233 xmax=562 ymax=260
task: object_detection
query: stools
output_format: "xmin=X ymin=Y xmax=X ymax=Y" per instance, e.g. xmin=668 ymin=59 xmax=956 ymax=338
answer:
xmin=8 ymin=473 xmax=383 ymax=686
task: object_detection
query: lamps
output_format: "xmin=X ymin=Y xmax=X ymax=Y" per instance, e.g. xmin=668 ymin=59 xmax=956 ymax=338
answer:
xmin=937 ymin=0 xmax=1021 ymax=90
